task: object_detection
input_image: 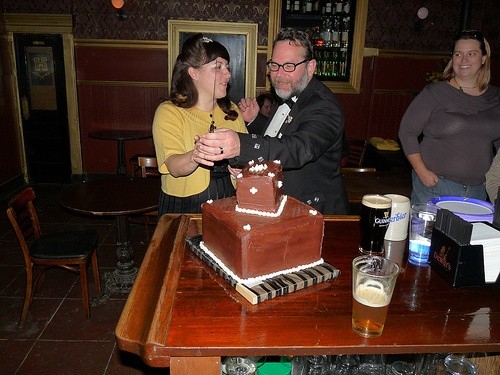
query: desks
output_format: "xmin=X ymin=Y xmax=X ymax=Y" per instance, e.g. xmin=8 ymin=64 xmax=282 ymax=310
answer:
xmin=57 ymin=176 xmax=161 ymax=308
xmin=89 ymin=129 xmax=152 ymax=174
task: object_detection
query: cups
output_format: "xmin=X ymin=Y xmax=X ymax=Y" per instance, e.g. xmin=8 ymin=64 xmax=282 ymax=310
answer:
xmin=352 ymin=254 xmax=400 ymax=338
xmin=357 ymin=194 xmax=392 ymax=256
xmin=407 ymin=203 xmax=442 ymax=266
xmin=383 ymin=194 xmax=410 ymax=241
xmin=291 ymin=352 xmax=478 ymax=375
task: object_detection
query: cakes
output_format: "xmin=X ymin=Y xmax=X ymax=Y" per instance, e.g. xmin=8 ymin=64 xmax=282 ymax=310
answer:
xmin=199 ymin=156 xmax=325 ymax=282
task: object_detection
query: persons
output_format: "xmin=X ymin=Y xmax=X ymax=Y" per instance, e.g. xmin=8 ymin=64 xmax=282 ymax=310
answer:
xmin=198 ymin=28 xmax=347 ymax=215
xmin=397 ymin=29 xmax=500 ymax=205
xmin=153 ymin=33 xmax=249 ymax=215
xmin=256 ymin=93 xmax=276 ymax=118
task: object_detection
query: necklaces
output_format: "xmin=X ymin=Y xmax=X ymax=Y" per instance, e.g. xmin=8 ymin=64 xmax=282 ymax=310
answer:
xmin=454 ymin=76 xmax=477 ymax=94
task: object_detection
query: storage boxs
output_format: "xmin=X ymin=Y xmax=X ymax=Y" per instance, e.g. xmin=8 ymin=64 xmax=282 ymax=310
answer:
xmin=428 ymin=219 xmax=500 ymax=288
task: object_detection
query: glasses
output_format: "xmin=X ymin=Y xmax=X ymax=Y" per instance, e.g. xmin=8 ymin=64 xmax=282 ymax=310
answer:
xmin=455 ymin=31 xmax=484 ymax=54
xmin=266 ymin=57 xmax=312 ymax=72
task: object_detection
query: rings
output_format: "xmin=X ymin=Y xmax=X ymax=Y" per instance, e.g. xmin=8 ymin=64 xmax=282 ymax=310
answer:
xmin=220 ymin=147 xmax=223 ymax=154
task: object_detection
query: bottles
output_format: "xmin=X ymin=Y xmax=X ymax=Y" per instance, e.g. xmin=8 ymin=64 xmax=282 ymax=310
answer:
xmin=307 ymin=16 xmax=350 ymax=47
xmin=315 ymin=48 xmax=347 ymax=77
xmin=284 ymin=0 xmax=351 ymax=17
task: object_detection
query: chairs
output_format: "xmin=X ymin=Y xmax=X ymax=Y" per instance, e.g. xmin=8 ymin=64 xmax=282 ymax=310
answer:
xmin=7 ymin=188 xmax=100 ymax=330
xmin=136 ymin=156 xmax=158 ymax=177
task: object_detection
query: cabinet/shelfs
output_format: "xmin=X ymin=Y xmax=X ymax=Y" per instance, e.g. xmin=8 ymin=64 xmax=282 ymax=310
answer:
xmin=266 ymin=0 xmax=367 ymax=94
xmin=112 ymin=212 xmax=500 ymax=375
